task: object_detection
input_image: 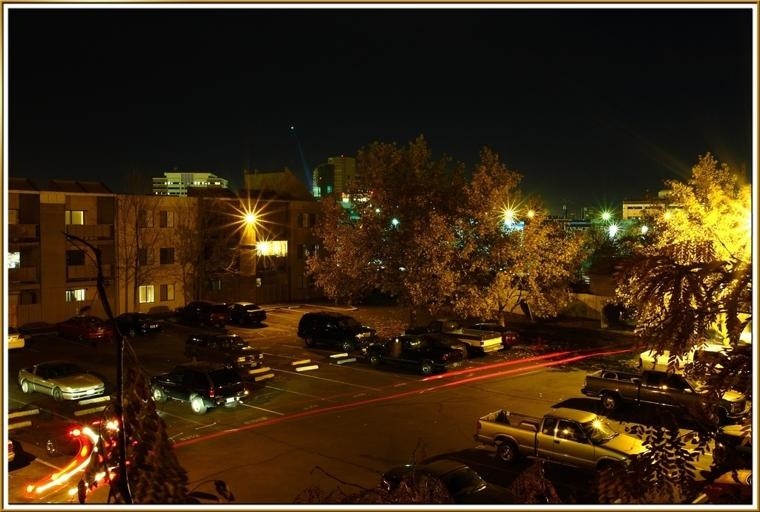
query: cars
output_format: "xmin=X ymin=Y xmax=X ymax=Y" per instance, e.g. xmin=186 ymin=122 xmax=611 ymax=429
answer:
xmin=60 ymin=296 xmax=268 ymax=413
xmin=294 ymin=310 xmax=520 ymax=374
xmin=7 ymin=438 xmax=16 ymax=462
xmin=8 ymin=324 xmax=26 ymax=354
xmin=19 ymin=358 xmax=105 ymax=404
